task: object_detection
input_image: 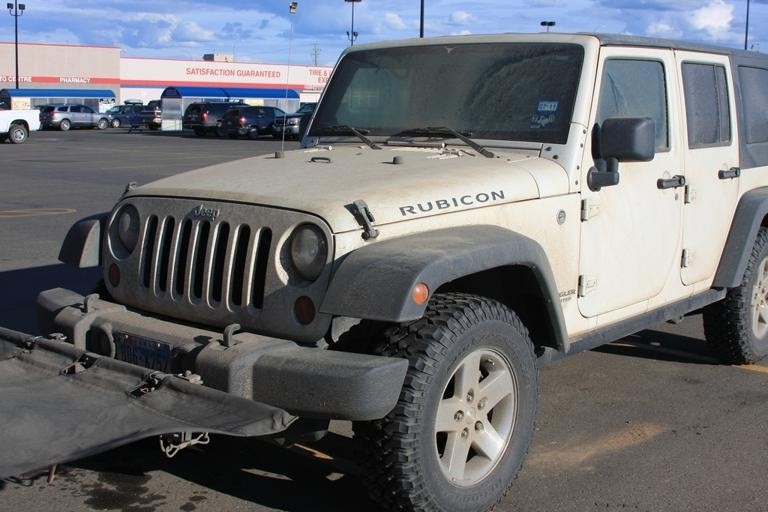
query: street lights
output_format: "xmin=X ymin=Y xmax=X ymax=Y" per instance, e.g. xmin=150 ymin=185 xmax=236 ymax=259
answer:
xmin=540 ymin=21 xmax=556 ymax=32
xmin=344 ymin=0 xmax=362 ymax=46
xmin=7 ymin=0 xmax=25 ymax=89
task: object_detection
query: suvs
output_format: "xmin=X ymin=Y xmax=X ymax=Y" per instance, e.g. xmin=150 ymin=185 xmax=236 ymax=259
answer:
xmin=36 ymin=31 xmax=768 ymax=512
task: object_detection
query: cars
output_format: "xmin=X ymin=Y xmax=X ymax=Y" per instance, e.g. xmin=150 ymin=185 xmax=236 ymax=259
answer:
xmin=183 ymin=102 xmax=317 ymax=139
xmin=41 ymin=99 xmax=161 ymax=132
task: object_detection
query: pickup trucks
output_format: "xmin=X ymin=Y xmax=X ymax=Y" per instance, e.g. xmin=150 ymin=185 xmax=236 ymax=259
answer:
xmin=0 ymin=109 xmax=41 ymax=144
